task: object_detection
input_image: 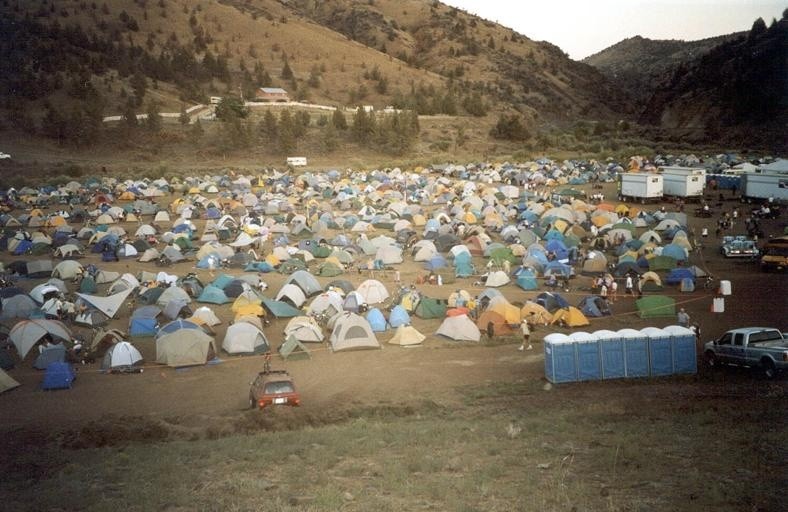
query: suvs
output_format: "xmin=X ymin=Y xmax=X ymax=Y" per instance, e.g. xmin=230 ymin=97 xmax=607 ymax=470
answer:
xmin=248 ymin=369 xmax=301 ymax=412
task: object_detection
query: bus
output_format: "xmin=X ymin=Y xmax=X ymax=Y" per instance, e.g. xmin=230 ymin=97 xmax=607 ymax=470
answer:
xmin=758 ymin=235 xmax=787 ymax=271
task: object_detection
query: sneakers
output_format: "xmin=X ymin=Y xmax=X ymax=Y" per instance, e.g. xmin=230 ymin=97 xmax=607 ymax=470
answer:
xmin=518 ymin=345 xmax=534 ymax=351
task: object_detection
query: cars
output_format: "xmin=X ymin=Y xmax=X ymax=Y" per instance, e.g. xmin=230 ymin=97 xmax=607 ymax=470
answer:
xmin=0 ymin=151 xmax=12 ymax=160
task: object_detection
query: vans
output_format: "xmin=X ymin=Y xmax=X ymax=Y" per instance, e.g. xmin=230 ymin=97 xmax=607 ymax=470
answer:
xmin=285 ymin=156 xmax=307 ymax=168
xmin=210 ymin=96 xmax=222 ymax=104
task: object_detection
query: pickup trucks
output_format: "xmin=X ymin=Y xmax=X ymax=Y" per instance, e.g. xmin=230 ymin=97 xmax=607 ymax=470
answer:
xmin=700 ymin=326 xmax=787 ymax=381
